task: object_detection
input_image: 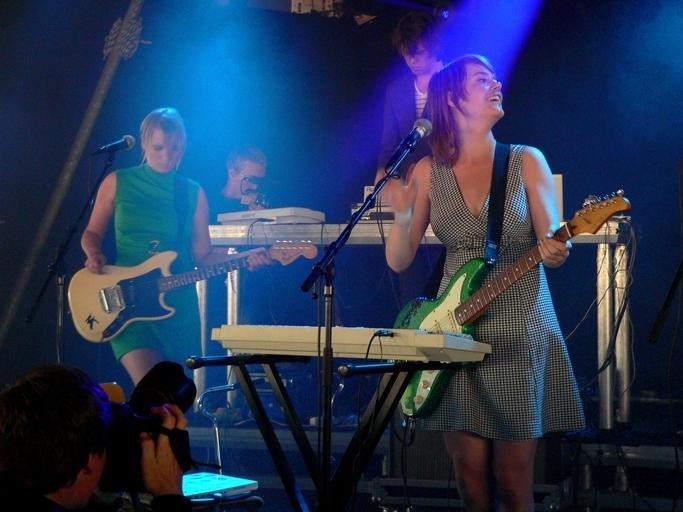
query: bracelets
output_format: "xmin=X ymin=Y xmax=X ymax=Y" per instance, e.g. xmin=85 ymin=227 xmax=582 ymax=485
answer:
xmin=392 ymin=207 xmax=412 ymax=223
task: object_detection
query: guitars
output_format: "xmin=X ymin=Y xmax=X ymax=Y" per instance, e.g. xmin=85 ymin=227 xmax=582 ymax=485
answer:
xmin=66 ymin=235 xmax=319 ymax=343
xmin=389 ymin=188 xmax=631 ymax=418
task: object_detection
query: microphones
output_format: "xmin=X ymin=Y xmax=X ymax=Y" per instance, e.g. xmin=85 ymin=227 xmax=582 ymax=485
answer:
xmin=92 ymin=134 xmax=137 ymax=157
xmin=384 ymin=118 xmax=434 ymax=170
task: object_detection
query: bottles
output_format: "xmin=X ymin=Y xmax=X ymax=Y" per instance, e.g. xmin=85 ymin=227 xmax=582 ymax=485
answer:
xmin=580 ymin=465 xmax=591 ymax=491
xmin=615 ymin=466 xmax=631 ymax=493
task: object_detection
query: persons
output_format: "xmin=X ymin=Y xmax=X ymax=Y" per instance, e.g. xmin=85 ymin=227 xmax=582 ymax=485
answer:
xmin=375 ymin=52 xmax=588 ymax=511
xmin=375 ymin=12 xmax=451 ymax=301
xmin=79 ymin=104 xmax=275 ymax=389
xmin=208 ymin=147 xmax=268 ymax=219
xmin=0 ymin=362 xmax=191 ymax=510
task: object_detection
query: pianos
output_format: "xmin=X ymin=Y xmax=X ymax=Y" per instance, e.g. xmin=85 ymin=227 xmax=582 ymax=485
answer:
xmin=210 ymin=323 xmax=492 ymax=370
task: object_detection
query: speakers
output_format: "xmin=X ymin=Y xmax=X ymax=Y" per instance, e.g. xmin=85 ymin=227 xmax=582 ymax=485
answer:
xmin=390 ymin=408 xmax=547 ymax=483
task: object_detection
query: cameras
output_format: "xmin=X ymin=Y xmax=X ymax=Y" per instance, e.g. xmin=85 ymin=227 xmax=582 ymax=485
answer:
xmin=110 ymin=359 xmax=197 ymax=472
xmin=240 ymin=165 xmax=281 ymax=208
